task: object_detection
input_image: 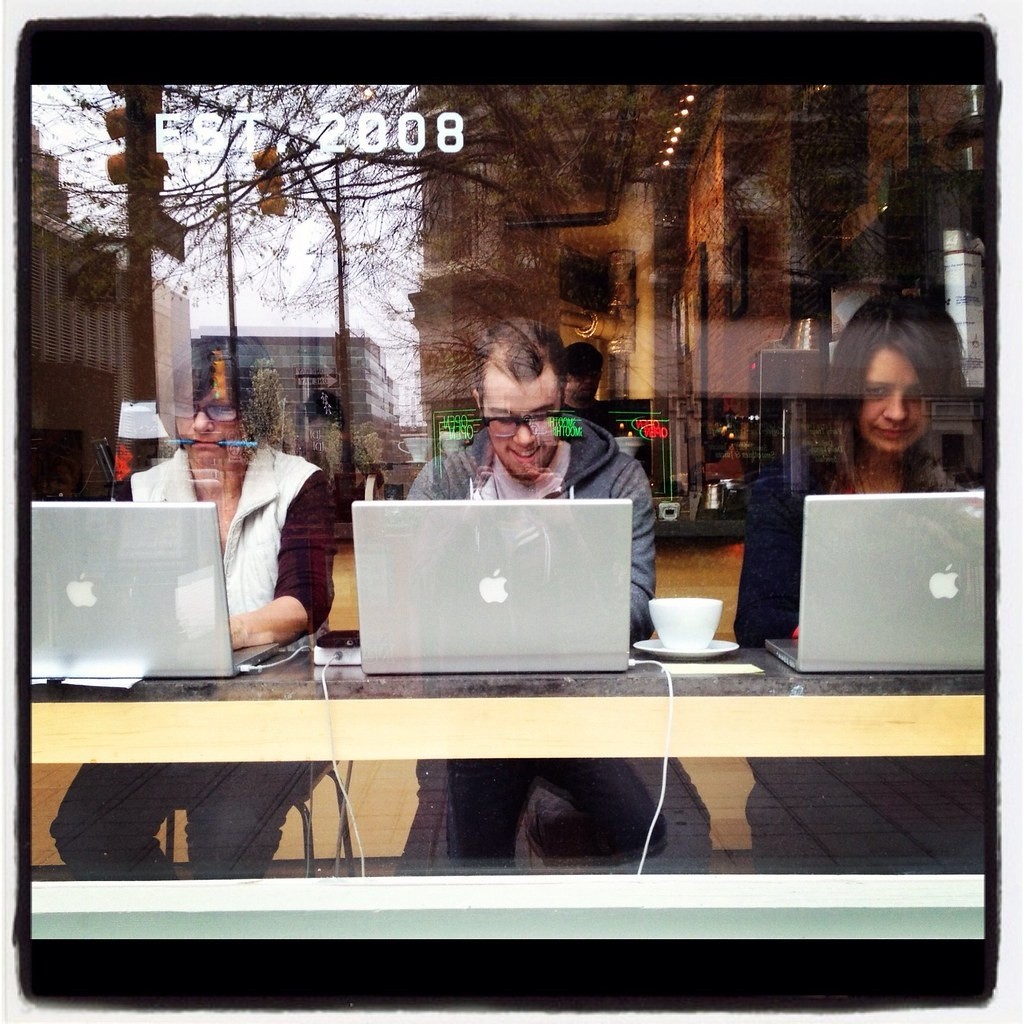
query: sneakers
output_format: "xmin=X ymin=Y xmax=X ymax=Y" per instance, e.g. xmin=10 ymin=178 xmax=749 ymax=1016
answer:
xmin=526 ymin=798 xmax=576 ymax=867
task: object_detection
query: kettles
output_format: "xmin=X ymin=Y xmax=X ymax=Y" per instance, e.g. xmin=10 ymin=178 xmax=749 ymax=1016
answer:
xmin=791 ymin=317 xmax=817 ymax=351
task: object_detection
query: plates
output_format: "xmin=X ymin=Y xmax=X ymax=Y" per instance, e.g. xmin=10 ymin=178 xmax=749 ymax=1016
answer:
xmin=633 ymin=639 xmax=741 ymax=662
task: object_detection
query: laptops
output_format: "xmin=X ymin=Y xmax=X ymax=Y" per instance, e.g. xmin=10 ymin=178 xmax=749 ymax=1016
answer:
xmin=351 ymin=498 xmax=633 ymax=674
xmin=766 ymin=492 xmax=987 ymax=673
xmin=29 ymin=499 xmax=278 ymax=676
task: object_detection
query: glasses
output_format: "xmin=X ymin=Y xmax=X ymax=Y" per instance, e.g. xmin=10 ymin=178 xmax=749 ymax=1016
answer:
xmin=482 ymin=412 xmax=562 ymax=437
xmin=167 ymin=401 xmax=248 ymax=422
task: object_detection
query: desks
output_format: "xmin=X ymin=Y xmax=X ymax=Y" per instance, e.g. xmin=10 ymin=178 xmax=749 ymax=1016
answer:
xmin=31 ymin=626 xmax=989 ymax=758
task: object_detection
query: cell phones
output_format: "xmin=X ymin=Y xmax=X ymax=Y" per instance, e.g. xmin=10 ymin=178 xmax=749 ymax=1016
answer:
xmin=316 ymin=630 xmax=363 ymax=649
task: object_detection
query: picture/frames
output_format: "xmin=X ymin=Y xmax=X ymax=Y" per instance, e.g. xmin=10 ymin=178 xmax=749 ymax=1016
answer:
xmin=676 ymin=242 xmax=707 ymax=365
xmin=724 ymin=227 xmax=748 ymax=322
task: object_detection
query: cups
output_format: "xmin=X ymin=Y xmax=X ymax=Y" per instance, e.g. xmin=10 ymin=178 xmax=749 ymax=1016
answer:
xmin=647 ymin=598 xmax=723 ymax=653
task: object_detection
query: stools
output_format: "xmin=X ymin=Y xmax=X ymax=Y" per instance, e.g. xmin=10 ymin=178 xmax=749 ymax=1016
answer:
xmin=164 ymin=761 xmax=356 ymax=878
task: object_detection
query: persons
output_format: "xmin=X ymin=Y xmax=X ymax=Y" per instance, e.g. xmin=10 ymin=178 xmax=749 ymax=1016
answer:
xmin=298 ymin=392 xmax=385 ymax=520
xmin=49 ymin=324 xmax=334 ymax=877
xmin=740 ymin=296 xmax=987 ymax=868
xmin=398 ymin=316 xmax=710 ymax=871
xmin=562 ymin=343 xmax=619 ymax=437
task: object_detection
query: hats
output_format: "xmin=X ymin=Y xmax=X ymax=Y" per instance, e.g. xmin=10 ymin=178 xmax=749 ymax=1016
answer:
xmin=566 ymin=343 xmax=604 ymax=375
